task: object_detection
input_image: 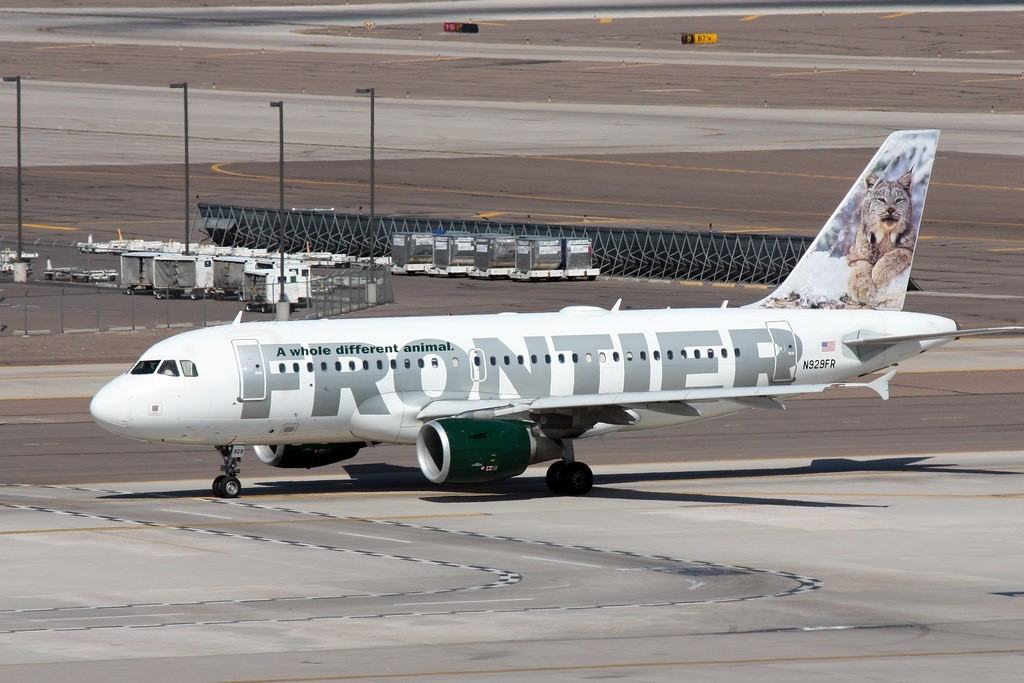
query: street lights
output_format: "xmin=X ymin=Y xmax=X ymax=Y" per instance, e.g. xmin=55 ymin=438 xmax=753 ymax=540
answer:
xmin=269 ymin=101 xmax=292 ymax=319
xmin=169 ymin=82 xmax=200 ymax=257
xmin=3 ymin=75 xmax=26 ymax=285
xmin=354 ymin=88 xmax=382 ymax=302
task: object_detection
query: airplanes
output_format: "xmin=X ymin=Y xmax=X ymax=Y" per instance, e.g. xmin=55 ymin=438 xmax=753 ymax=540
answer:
xmin=89 ymin=128 xmax=1024 ymax=503
xmin=44 ymin=256 xmax=89 ymax=282
